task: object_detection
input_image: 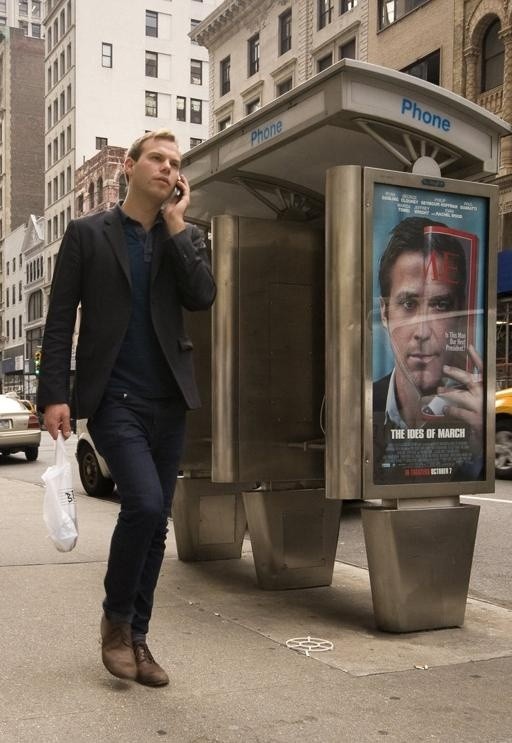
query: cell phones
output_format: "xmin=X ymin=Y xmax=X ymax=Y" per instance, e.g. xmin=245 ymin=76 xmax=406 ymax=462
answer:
xmin=171 ymin=171 xmax=182 ymax=201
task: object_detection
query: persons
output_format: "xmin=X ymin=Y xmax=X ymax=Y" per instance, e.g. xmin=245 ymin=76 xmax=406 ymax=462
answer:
xmin=372 ymin=217 xmax=486 ymax=481
xmin=37 ymin=130 xmax=217 ymax=685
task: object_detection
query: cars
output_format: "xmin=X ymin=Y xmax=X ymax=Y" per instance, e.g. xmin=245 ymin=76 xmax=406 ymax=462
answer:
xmin=362 ymin=287 xmax=485 ymax=442
xmin=19 ymin=399 xmax=35 ymax=414
xmin=75 ymin=417 xmax=113 ymax=496
xmin=0 ymin=394 xmax=42 ymax=465
xmin=494 ymin=383 xmax=512 ymax=479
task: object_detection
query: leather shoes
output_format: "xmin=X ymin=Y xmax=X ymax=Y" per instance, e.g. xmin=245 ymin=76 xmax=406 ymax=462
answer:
xmin=131 ymin=637 xmax=170 ymax=686
xmin=101 ymin=613 xmax=137 ymax=680
